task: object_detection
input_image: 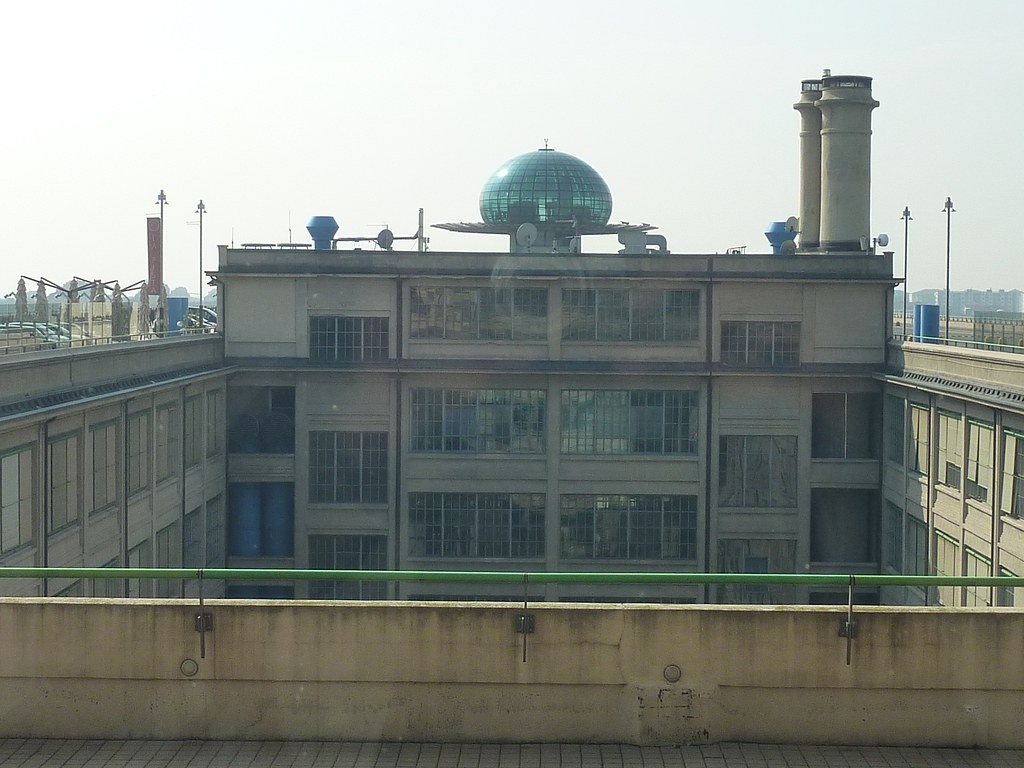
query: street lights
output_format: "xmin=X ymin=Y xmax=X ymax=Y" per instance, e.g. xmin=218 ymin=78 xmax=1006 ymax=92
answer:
xmin=941 ymin=196 xmax=956 ymax=344
xmin=899 ymin=206 xmax=913 ymax=341
xmin=155 ymin=190 xmax=169 ymax=337
xmin=195 ymin=199 xmax=208 ymax=328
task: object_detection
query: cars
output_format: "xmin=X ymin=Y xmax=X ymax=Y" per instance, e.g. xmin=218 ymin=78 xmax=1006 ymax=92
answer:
xmin=187 ymin=306 xmax=217 ymax=327
xmin=0 ymin=322 xmax=71 ymax=349
